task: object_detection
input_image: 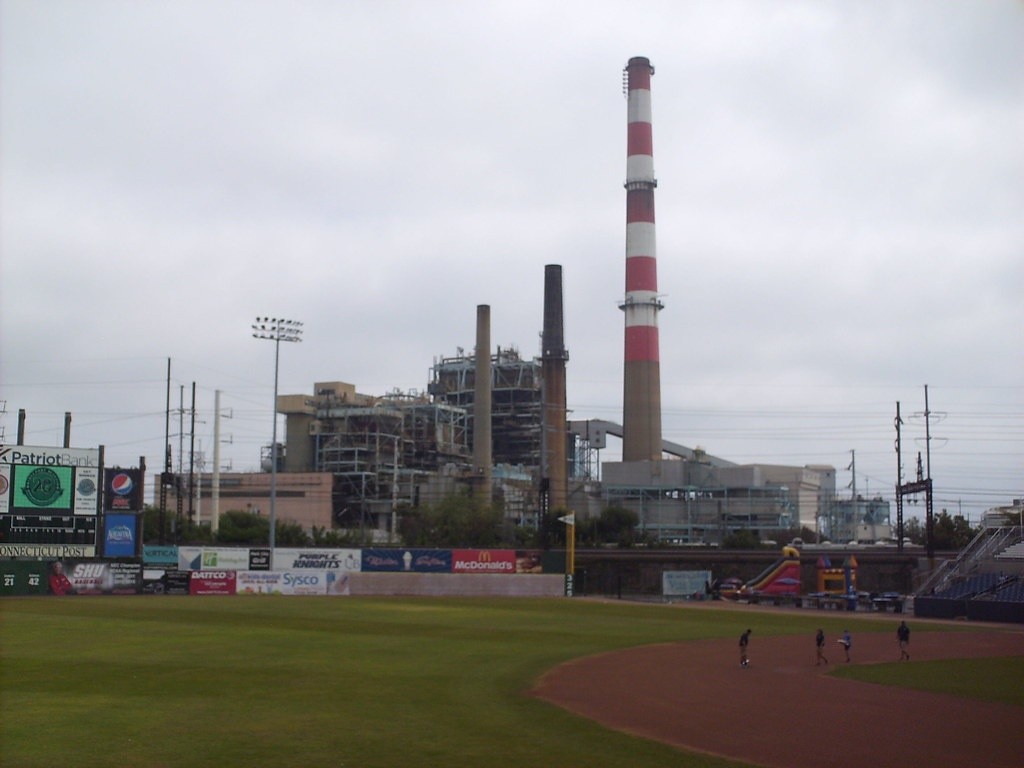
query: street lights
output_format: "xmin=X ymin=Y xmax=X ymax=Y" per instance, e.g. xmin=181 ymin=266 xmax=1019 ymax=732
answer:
xmin=251 ymin=315 xmax=305 ymax=571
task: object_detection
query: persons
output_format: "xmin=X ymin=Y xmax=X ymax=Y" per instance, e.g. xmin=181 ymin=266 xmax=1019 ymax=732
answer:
xmin=48 ymin=562 xmax=71 ymax=596
xmin=739 ymin=629 xmax=752 ymax=668
xmin=842 ymin=630 xmax=851 ymax=663
xmin=896 ymin=621 xmax=913 ymax=659
xmin=814 ymin=628 xmax=829 ymax=667
